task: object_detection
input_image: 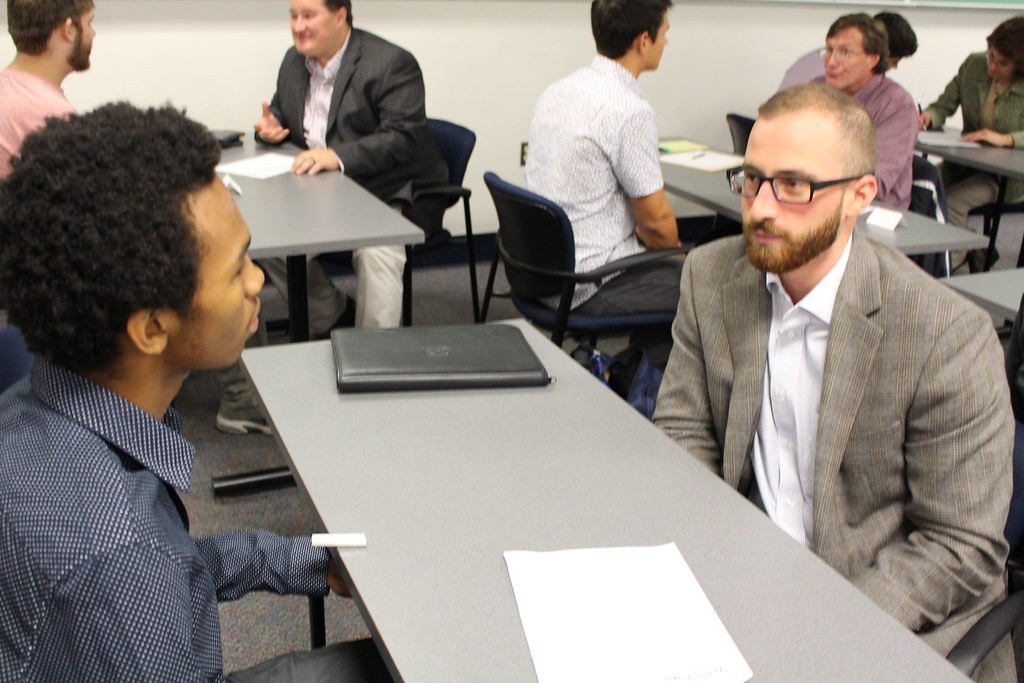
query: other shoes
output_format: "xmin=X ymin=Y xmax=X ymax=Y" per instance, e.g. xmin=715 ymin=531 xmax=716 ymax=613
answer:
xmin=314 ymin=295 xmax=356 ymax=340
xmin=214 ymin=409 xmax=272 ymax=439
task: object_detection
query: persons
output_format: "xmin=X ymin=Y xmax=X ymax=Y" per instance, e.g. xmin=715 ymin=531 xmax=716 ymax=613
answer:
xmin=653 ymin=84 xmax=1019 ymax=683
xmin=522 ymin=0 xmax=686 ymax=371
xmin=773 ymin=10 xmax=1024 ymax=275
xmin=0 ymin=0 xmax=274 ymax=434
xmin=255 ymin=0 xmax=449 ymax=340
xmin=0 ymin=101 xmax=393 ymax=683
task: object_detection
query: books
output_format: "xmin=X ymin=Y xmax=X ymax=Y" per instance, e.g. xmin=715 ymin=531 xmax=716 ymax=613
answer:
xmin=331 ymin=324 xmax=550 ymax=393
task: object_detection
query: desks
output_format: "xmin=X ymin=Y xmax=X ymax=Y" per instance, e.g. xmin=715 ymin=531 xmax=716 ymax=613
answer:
xmin=914 ymin=125 xmax=1024 ymax=268
xmin=938 ymin=267 xmax=1024 ymax=322
xmin=239 ymin=317 xmax=979 ymax=683
xmin=211 ymin=130 xmax=425 ymax=496
xmin=659 ymin=161 xmax=990 ymax=278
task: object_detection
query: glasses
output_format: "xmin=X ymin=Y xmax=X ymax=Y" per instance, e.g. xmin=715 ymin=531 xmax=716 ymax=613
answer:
xmin=820 ymin=43 xmax=867 ymax=65
xmin=726 ymin=165 xmax=874 ymax=206
xmin=984 ymin=45 xmax=1016 ymax=77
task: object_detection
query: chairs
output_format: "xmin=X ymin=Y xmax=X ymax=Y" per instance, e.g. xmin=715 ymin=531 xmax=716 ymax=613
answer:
xmin=401 ymin=113 xmax=1024 ymax=683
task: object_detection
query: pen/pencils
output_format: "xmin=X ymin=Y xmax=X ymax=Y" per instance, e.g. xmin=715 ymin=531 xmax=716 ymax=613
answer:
xmin=918 ymin=103 xmax=926 ymax=131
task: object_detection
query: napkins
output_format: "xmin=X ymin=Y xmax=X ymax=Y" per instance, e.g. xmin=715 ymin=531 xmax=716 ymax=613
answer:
xmin=312 ymin=532 xmax=366 ymax=548
xmin=213 ymin=152 xmax=298 ymax=180
xmin=501 ymin=541 xmax=754 ymax=683
